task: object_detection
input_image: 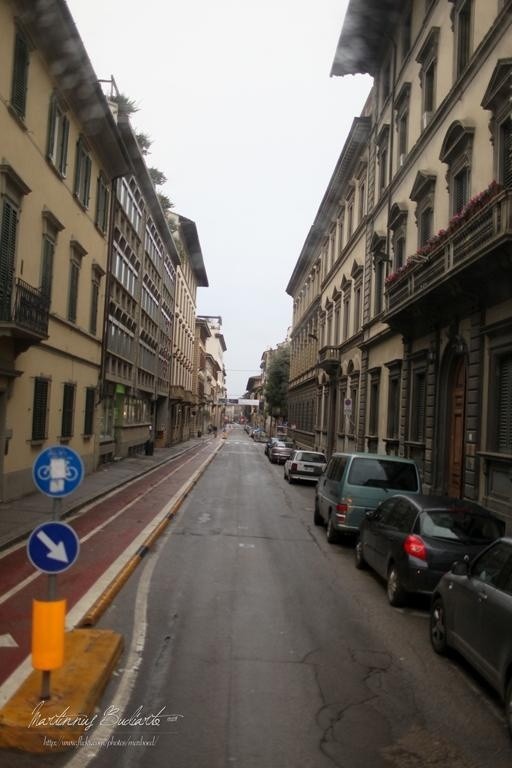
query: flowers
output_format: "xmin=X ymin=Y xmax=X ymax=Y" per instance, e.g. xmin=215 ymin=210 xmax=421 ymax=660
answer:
xmin=384 ymin=180 xmax=501 ymax=288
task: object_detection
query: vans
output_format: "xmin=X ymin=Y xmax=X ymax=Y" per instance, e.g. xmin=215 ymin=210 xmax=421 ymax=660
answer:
xmin=313 ymin=452 xmax=423 ymax=544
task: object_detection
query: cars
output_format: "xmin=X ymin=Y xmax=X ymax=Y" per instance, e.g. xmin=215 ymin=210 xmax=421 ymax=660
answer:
xmin=428 ymin=535 xmax=512 ymax=744
xmin=354 ymin=493 xmax=503 ymax=608
xmin=283 ymin=449 xmax=329 ymax=484
xmin=265 ymin=437 xmax=298 ymax=464
